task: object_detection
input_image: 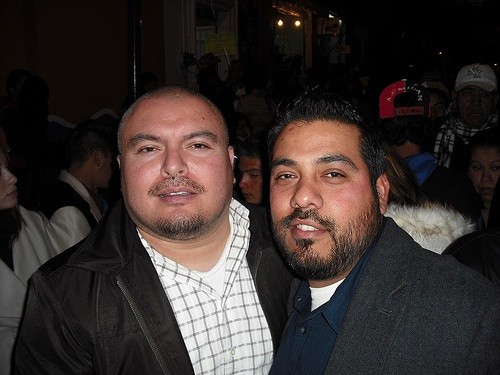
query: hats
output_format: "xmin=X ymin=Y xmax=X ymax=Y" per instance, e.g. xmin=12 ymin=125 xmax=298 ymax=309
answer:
xmin=379 ymin=78 xmax=430 ymax=120
xmin=455 ymin=64 xmax=497 ymax=93
xmin=420 ymin=80 xmax=450 ymax=101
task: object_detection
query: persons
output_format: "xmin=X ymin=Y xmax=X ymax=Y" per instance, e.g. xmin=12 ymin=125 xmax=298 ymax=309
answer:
xmin=0 ymin=48 xmax=500 ymax=285
xmin=0 ymin=125 xmax=71 ymax=375
xmin=267 ymin=88 xmax=500 ymax=375
xmin=10 ymin=86 xmax=300 ymax=375
